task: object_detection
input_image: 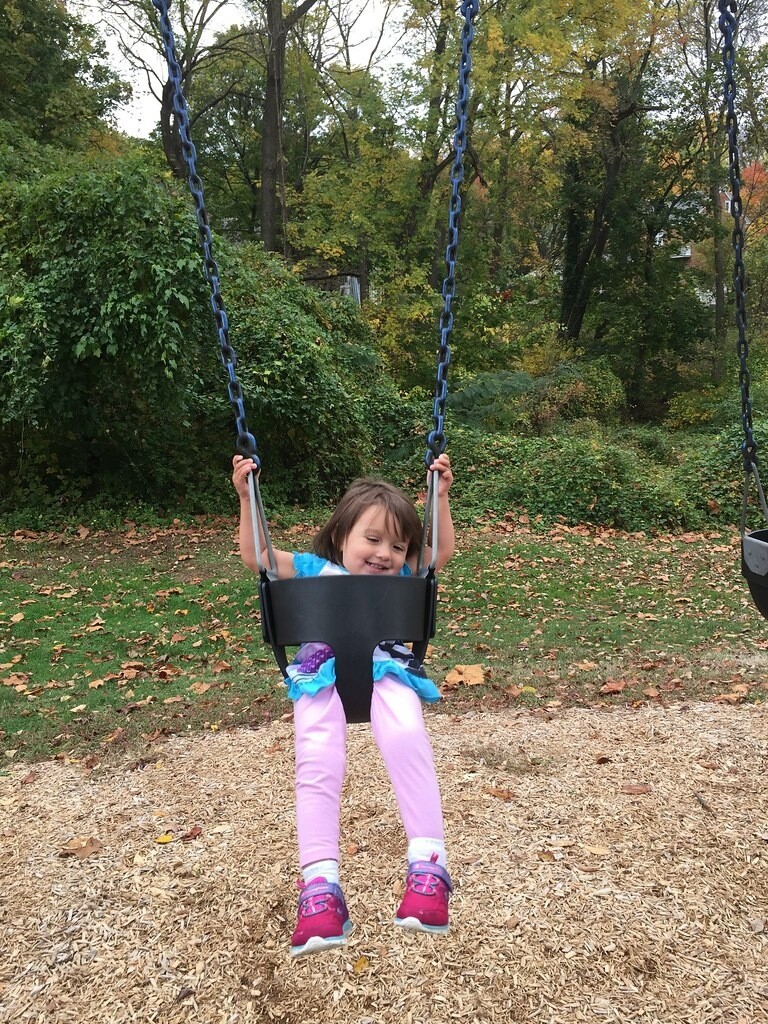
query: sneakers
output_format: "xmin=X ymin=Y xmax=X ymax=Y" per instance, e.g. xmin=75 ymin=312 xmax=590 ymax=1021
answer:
xmin=289 ymin=876 xmax=352 ymax=959
xmin=394 ymin=853 xmax=453 ymax=934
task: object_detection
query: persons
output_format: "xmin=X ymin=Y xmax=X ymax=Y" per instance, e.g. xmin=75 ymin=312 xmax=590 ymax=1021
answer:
xmin=232 ymin=455 xmax=454 ymax=955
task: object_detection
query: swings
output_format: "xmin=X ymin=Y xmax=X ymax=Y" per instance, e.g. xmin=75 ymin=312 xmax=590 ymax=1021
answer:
xmin=716 ymin=0 xmax=768 ymax=621
xmin=154 ymin=0 xmax=482 ymax=724
